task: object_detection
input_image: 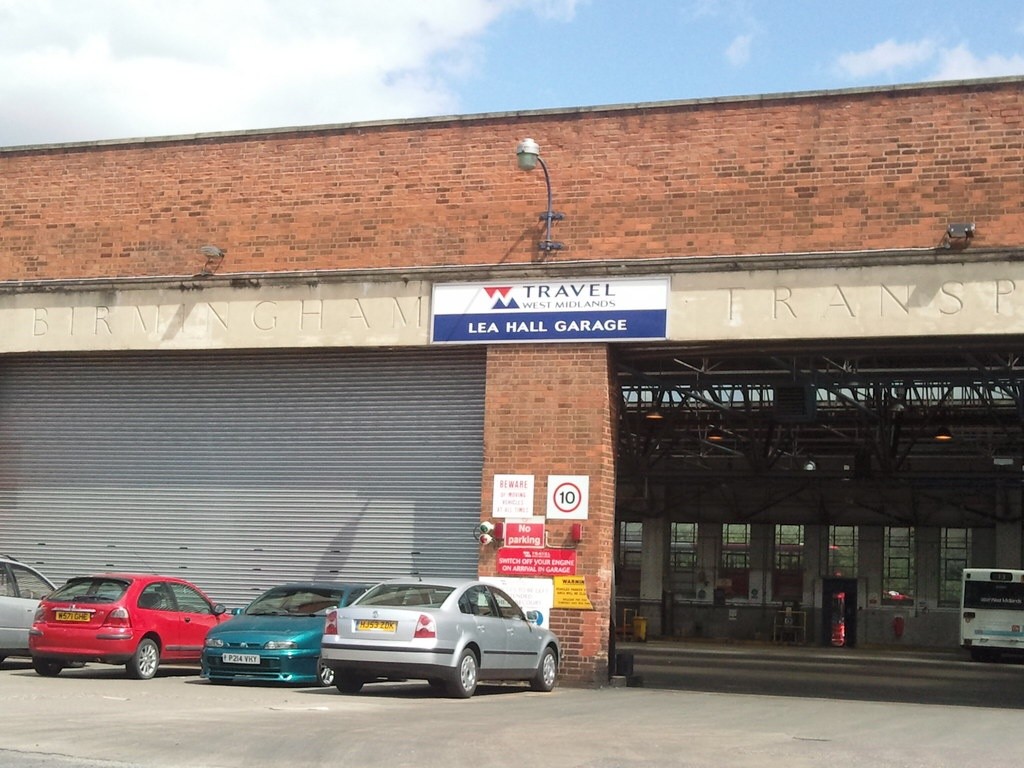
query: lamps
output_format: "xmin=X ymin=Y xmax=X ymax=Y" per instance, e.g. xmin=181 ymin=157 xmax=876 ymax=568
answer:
xmin=517 ymin=136 xmax=542 ymax=170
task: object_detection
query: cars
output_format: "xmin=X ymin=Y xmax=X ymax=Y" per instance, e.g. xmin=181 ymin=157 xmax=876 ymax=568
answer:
xmin=1 ymin=553 xmax=87 ymax=668
xmin=29 ymin=574 xmax=233 ymax=680
xmin=200 ymin=582 xmax=406 ymax=687
xmin=321 ymin=576 xmax=561 ymax=699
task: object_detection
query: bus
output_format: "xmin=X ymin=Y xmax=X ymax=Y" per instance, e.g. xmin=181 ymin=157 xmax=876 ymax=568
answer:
xmin=960 ymin=568 xmax=1024 ymax=658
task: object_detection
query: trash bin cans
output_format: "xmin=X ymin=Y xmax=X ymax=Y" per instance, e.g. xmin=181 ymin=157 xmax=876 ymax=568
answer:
xmin=632 ymin=616 xmax=645 ymax=642
